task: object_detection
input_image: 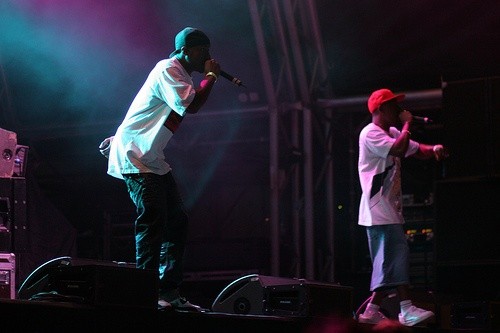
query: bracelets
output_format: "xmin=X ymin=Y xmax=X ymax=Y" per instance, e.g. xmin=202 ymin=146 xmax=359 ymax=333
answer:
xmin=206 ymin=72 xmax=217 ymax=80
xmin=402 ymin=130 xmax=412 ymax=135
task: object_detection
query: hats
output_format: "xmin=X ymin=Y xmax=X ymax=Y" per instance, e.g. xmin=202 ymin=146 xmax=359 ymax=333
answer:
xmin=169 ymin=26 xmax=211 ymax=58
xmin=367 ymin=89 xmax=405 ymax=114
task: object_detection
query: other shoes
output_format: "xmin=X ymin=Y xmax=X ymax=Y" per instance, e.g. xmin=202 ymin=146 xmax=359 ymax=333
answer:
xmin=158 ymin=297 xmax=201 ymax=309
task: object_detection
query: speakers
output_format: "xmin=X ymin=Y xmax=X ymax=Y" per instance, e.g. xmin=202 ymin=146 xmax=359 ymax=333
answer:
xmin=0 ymin=129 xmax=18 ymax=177
xmin=0 ymin=178 xmax=42 ymax=254
xmin=17 ymin=253 xmax=140 ymax=300
xmin=211 ymin=273 xmax=500 ymax=330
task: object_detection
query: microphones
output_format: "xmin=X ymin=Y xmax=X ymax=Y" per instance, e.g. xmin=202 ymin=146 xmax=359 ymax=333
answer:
xmin=219 ymin=69 xmax=243 ymax=86
xmin=411 ymin=115 xmax=434 ymax=123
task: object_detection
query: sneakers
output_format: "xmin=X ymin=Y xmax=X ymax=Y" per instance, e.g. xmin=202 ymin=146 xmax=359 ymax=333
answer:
xmin=358 ymin=311 xmax=389 ymax=324
xmin=398 ymin=305 xmax=434 ymax=327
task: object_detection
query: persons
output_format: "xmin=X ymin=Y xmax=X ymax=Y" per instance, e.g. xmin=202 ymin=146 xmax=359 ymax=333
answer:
xmin=98 ymin=26 xmax=221 ymax=312
xmin=357 ymin=89 xmax=448 ymax=327
